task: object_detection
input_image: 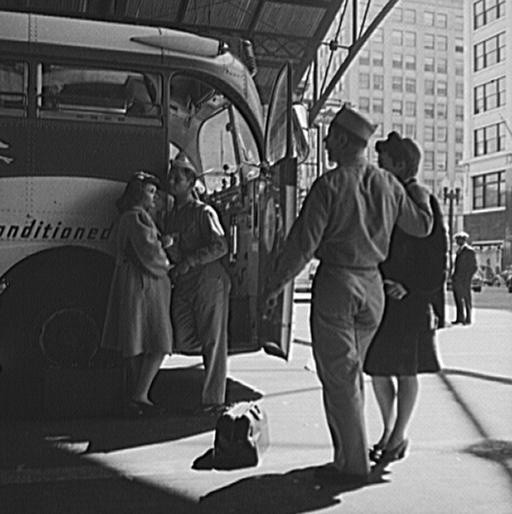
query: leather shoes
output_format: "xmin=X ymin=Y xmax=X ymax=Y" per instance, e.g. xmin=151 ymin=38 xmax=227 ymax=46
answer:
xmin=129 ymin=396 xmax=167 ymax=416
xmin=193 ymin=402 xmax=225 ymax=418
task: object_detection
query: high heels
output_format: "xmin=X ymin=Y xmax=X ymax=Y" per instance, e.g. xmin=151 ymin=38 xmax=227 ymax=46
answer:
xmin=367 ymin=438 xmax=409 ymax=464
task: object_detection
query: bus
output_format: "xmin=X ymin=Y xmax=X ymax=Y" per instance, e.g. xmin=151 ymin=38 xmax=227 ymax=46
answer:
xmin=1 ymin=9 xmax=311 ymax=409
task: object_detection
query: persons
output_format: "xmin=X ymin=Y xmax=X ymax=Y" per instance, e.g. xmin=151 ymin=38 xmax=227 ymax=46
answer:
xmin=162 ymin=162 xmax=231 ymax=417
xmin=257 ymin=102 xmax=433 ymax=486
xmin=451 ymin=231 xmax=477 ymax=324
xmin=361 ymin=131 xmax=447 ymax=469
xmin=99 ymin=170 xmax=173 ymax=411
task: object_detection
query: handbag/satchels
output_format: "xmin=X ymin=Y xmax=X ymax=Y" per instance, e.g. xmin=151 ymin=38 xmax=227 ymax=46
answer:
xmin=211 ymin=399 xmax=273 ymax=470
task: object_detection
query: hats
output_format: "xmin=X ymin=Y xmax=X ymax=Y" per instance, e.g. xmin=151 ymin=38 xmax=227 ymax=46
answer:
xmin=169 ymin=158 xmax=199 ymax=177
xmin=386 ymin=126 xmax=423 ymax=178
xmin=452 ymin=231 xmax=469 ymax=239
xmin=330 ymin=99 xmax=379 ymax=147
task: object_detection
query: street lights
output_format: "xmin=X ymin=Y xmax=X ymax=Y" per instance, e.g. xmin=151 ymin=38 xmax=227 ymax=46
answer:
xmin=442 ymin=184 xmax=459 ymax=278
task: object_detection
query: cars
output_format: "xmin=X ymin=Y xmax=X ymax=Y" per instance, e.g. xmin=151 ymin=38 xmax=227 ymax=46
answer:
xmin=295 ymin=257 xmax=321 ymax=294
xmin=445 ymin=257 xmax=512 ymax=292
